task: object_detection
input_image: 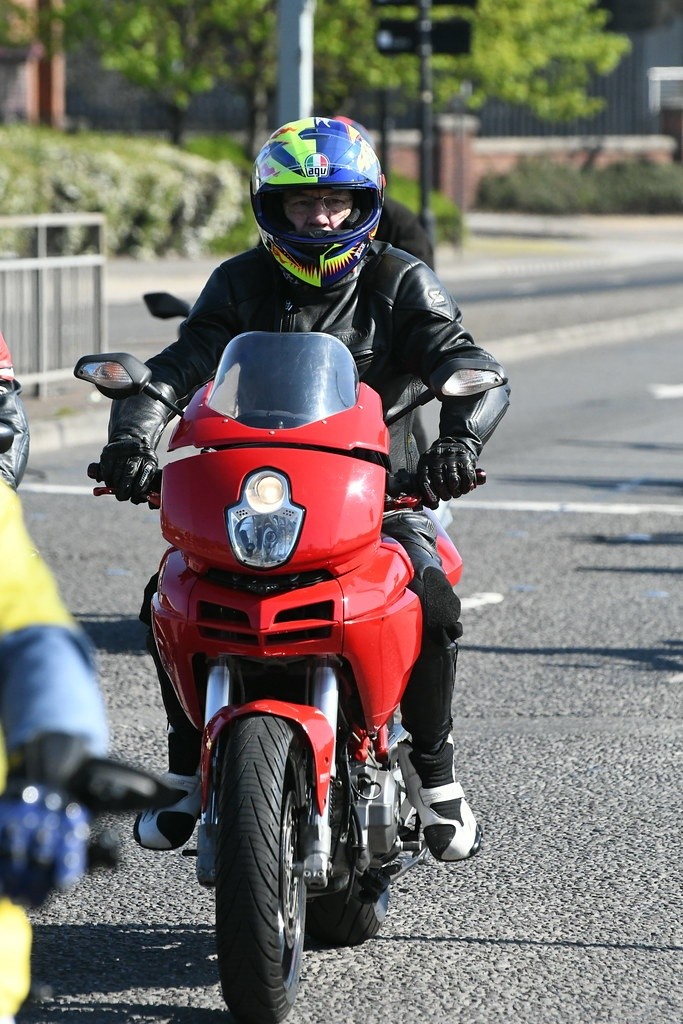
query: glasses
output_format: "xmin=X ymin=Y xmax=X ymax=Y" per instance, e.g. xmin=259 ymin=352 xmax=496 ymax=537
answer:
xmin=284 ymin=194 xmax=354 ymax=216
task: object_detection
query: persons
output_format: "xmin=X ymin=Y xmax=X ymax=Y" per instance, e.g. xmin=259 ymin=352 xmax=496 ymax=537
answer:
xmin=374 ymin=196 xmax=436 ymax=455
xmin=0 ymin=332 xmax=31 ymax=491
xmin=99 ymin=117 xmax=512 ymax=863
xmin=0 ymin=475 xmax=108 ymax=1017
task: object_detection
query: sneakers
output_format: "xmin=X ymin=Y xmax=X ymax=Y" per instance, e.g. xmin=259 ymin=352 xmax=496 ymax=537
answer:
xmin=133 ymin=722 xmax=203 ymax=851
xmin=409 ymin=733 xmax=482 ymax=863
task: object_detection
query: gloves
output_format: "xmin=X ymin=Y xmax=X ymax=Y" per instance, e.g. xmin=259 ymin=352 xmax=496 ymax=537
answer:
xmin=99 ymin=382 xmax=178 ymax=505
xmin=2 ymin=779 xmax=92 ymax=911
xmin=417 ymin=387 xmax=510 ymax=510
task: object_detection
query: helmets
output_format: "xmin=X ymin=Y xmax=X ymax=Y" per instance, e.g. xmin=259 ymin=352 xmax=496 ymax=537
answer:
xmin=250 ymin=116 xmax=387 ymax=287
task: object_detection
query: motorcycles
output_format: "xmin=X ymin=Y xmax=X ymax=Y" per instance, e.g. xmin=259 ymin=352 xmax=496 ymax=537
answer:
xmin=72 ymin=350 xmax=510 ymax=1024
